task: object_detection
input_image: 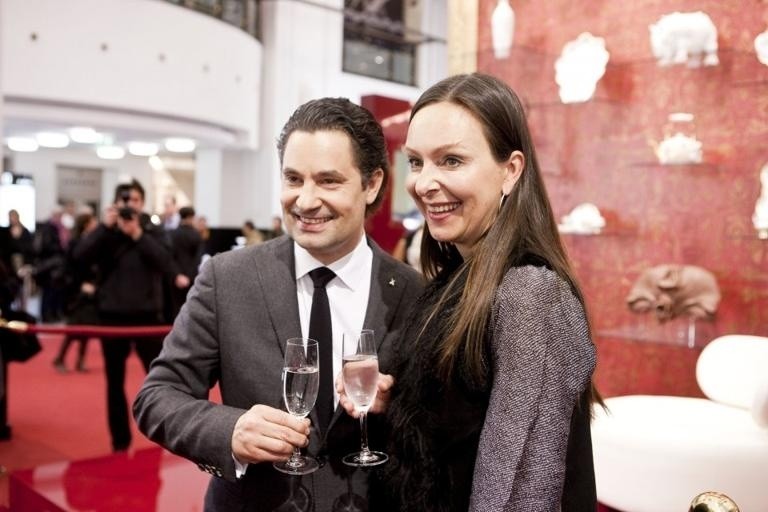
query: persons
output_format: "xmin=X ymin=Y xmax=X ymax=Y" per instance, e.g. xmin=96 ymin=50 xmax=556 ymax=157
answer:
xmin=0 ymin=179 xmax=286 ymax=452
xmin=393 ymin=223 xmax=423 ymax=268
xmin=323 ymin=74 xmax=602 ymax=512
xmin=129 ymin=94 xmax=437 ymax=512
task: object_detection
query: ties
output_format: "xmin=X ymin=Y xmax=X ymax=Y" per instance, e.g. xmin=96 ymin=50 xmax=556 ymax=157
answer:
xmin=307 ymin=267 xmax=337 ymax=438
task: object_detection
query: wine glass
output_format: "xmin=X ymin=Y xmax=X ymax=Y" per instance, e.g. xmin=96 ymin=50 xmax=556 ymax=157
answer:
xmin=340 ymin=327 xmax=389 ymax=470
xmin=271 ymin=336 xmax=320 ymax=477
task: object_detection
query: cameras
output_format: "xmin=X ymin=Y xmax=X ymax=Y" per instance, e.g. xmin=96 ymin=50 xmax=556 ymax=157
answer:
xmin=116 ymin=191 xmax=135 ymax=220
